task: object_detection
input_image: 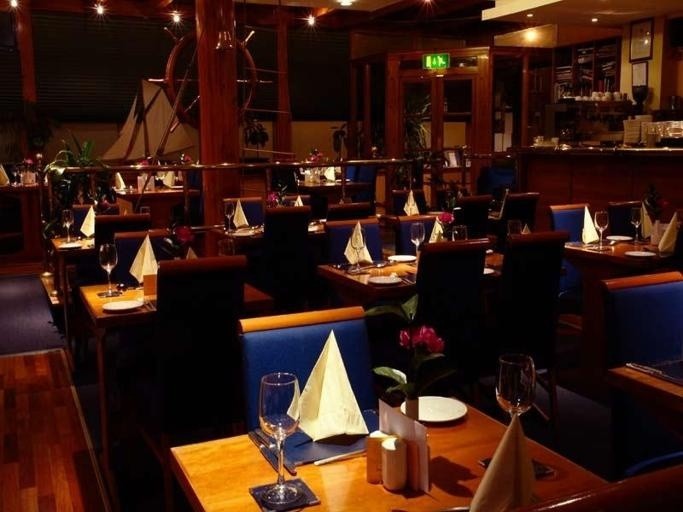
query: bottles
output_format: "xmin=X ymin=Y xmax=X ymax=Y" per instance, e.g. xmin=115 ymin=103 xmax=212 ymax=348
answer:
xmin=379 ymin=437 xmax=408 ymax=492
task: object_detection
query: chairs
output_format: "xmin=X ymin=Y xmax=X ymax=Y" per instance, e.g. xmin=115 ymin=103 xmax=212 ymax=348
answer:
xmin=477 ymin=230 xmax=566 ymax=432
xmin=157 ymin=255 xmax=248 ymax=325
xmin=68 ymin=190 xmax=683 ymax=350
xmin=596 ymin=270 xmax=682 ymax=459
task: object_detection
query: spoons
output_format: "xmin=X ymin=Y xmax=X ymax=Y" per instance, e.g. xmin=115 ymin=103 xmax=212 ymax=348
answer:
xmin=351 ymin=261 xmax=386 ymax=273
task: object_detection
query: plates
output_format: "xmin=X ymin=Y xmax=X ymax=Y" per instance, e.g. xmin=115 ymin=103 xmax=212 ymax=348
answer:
xmin=624 ymin=250 xmax=656 ymax=258
xmin=57 ymin=243 xmax=83 ymax=249
xmin=605 ymin=235 xmax=633 ymax=240
xmin=396 ymin=394 xmax=469 ymax=424
xmin=368 ymin=276 xmax=402 ymax=285
xmin=100 ymin=300 xmax=141 ymax=311
xmin=482 ymin=266 xmax=495 ymax=275
xmin=387 ymin=254 xmax=417 ymax=263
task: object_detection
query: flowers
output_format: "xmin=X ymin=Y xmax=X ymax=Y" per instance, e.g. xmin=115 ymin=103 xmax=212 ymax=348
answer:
xmin=397 ymin=324 xmax=445 ymax=363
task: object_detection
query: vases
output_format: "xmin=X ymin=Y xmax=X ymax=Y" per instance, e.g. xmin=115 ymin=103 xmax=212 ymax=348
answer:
xmin=378 ymin=399 xmax=432 ymax=491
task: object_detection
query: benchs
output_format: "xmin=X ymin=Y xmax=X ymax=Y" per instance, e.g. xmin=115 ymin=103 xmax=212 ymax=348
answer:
xmin=99 ymin=306 xmax=372 ymax=511
xmin=530 ymin=451 xmax=683 ymax=510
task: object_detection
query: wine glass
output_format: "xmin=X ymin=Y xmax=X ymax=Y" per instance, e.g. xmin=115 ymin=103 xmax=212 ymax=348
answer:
xmin=224 ymin=203 xmax=235 ymax=233
xmin=593 ymin=211 xmax=611 ymax=250
xmin=349 ymin=225 xmax=367 ymax=274
xmin=255 ymin=370 xmax=301 ymax=506
xmin=630 ymin=207 xmax=644 ymax=242
xmin=409 ymin=221 xmax=425 ymax=263
xmin=58 ymin=208 xmax=77 ymax=245
xmin=96 ymin=242 xmax=121 ymax=296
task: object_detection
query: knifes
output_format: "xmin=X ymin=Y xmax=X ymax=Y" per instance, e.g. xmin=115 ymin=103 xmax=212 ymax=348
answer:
xmin=247 ymin=430 xmax=297 ymax=477
xmin=142 ymin=299 xmax=156 ymax=312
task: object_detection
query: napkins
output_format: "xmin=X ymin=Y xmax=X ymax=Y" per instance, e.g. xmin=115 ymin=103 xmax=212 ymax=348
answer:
xmin=288 ymin=327 xmax=370 ymax=442
xmin=469 ymin=412 xmax=531 ymax=510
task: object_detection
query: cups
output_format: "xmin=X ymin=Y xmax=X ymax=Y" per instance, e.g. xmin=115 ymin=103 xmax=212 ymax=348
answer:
xmin=646 ymin=133 xmax=656 ymax=148
xmin=573 ymin=92 xmax=620 ymax=102
xmin=451 ymin=225 xmax=465 ymax=240
xmin=494 ymin=352 xmax=537 ymax=420
xmin=551 ymin=137 xmax=558 ymax=145
